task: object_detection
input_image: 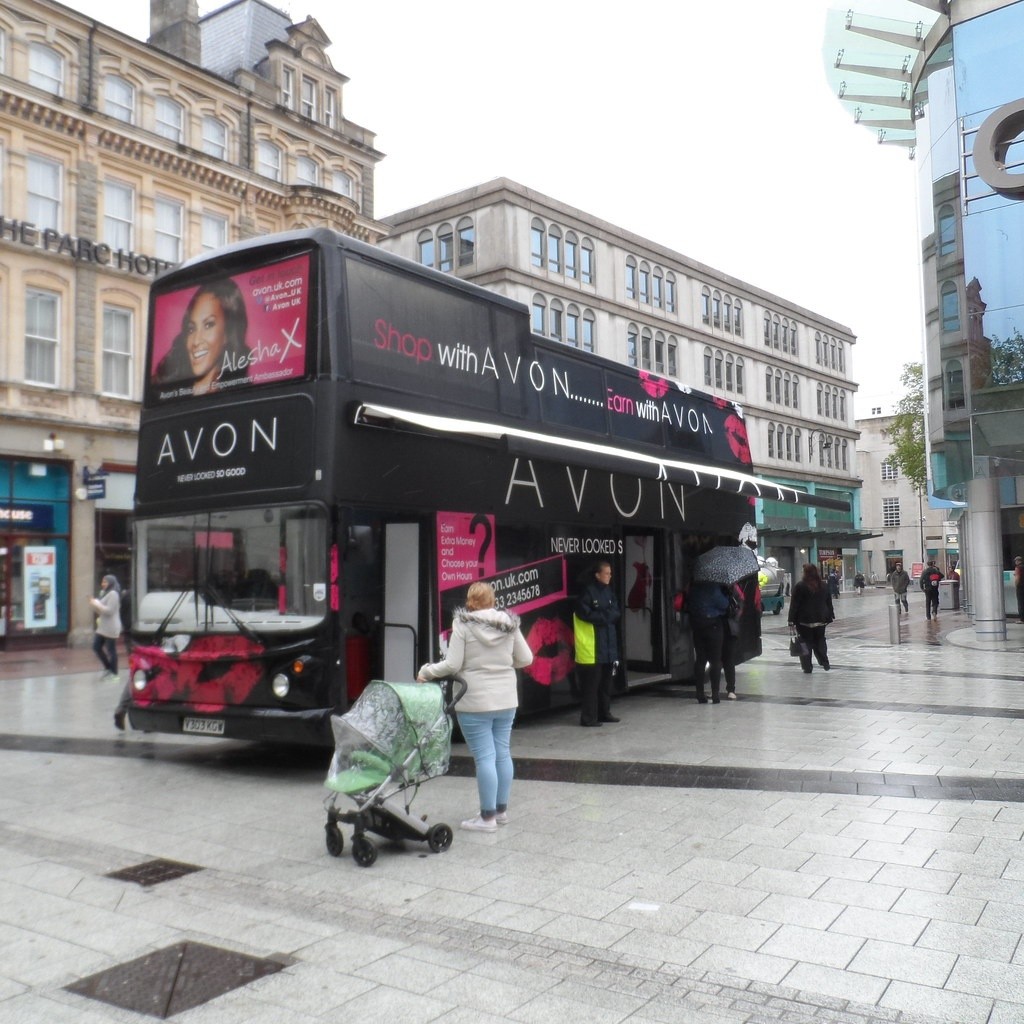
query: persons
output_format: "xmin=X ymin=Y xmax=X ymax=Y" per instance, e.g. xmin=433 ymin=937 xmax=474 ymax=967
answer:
xmin=707 ymin=583 xmax=745 ymax=700
xmin=947 ymin=566 xmax=960 ymax=609
xmin=687 ymin=577 xmax=730 ymax=704
xmin=788 ymin=563 xmax=835 ymax=673
xmin=87 ymin=574 xmax=122 ymax=683
xmin=854 ymin=570 xmax=865 ymax=594
xmin=870 ymin=571 xmax=878 ymax=590
xmin=832 ymin=571 xmax=840 ymax=598
xmin=891 ymin=563 xmax=910 ymax=614
xmin=150 ymin=278 xmax=252 ymax=402
xmin=574 ymin=562 xmax=621 ymax=727
xmin=416 ymin=581 xmax=533 ymax=833
xmin=826 ymin=570 xmax=838 ymax=599
xmin=1013 ymin=555 xmax=1024 ymax=624
xmin=920 ymin=561 xmax=945 ymax=621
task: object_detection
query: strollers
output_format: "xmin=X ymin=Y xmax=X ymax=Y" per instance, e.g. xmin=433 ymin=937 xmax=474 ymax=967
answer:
xmin=318 ymin=670 xmax=468 ymax=866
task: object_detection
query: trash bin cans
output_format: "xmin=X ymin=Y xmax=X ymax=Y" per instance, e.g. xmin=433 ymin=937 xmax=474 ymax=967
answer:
xmin=938 ymin=580 xmax=960 ymax=610
xmin=913 ymin=573 xmax=924 ymax=592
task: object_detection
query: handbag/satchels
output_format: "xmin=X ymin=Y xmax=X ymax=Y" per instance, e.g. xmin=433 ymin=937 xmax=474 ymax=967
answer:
xmin=790 ymin=626 xmax=809 ymax=656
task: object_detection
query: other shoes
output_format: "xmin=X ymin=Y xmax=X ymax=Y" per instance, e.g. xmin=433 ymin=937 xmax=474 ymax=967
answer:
xmin=99 ymin=669 xmax=111 ymax=679
xmin=898 ymin=609 xmax=901 ymax=614
xmin=728 ymin=692 xmax=736 ymax=698
xmin=927 ymin=617 xmax=931 ymax=621
xmin=712 ymin=689 xmax=720 ymax=703
xmin=696 ymin=687 xmax=708 ymax=703
xmin=804 ymin=669 xmax=812 ymax=673
xmin=597 ymin=713 xmax=620 ymax=722
xmin=1016 ymin=620 xmax=1024 ymax=624
xmin=905 ymin=608 xmax=908 ymax=612
xmin=110 ymin=674 xmax=120 ymax=680
xmin=591 ymin=722 xmax=602 ymax=726
xmin=933 ymin=613 xmax=937 ymax=622
xmin=824 ymin=656 xmax=830 ymax=671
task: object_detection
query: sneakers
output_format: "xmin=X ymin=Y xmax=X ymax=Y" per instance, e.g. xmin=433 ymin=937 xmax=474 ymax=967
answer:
xmin=461 ymin=812 xmax=497 ymax=832
xmin=477 ymin=812 xmax=510 ymax=824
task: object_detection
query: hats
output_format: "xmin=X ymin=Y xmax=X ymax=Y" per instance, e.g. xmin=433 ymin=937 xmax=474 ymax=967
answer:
xmin=896 ymin=563 xmax=901 ymax=565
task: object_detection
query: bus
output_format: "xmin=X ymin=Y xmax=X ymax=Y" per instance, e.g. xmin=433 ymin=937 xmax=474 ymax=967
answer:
xmin=115 ymin=232 xmax=764 ymax=740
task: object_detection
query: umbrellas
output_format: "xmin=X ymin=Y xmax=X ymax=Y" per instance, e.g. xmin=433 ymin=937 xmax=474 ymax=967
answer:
xmin=692 ymin=545 xmax=760 ymax=587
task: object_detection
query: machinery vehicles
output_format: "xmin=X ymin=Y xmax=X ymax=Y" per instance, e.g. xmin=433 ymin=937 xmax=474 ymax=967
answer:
xmin=755 ymin=555 xmax=786 ymax=615
